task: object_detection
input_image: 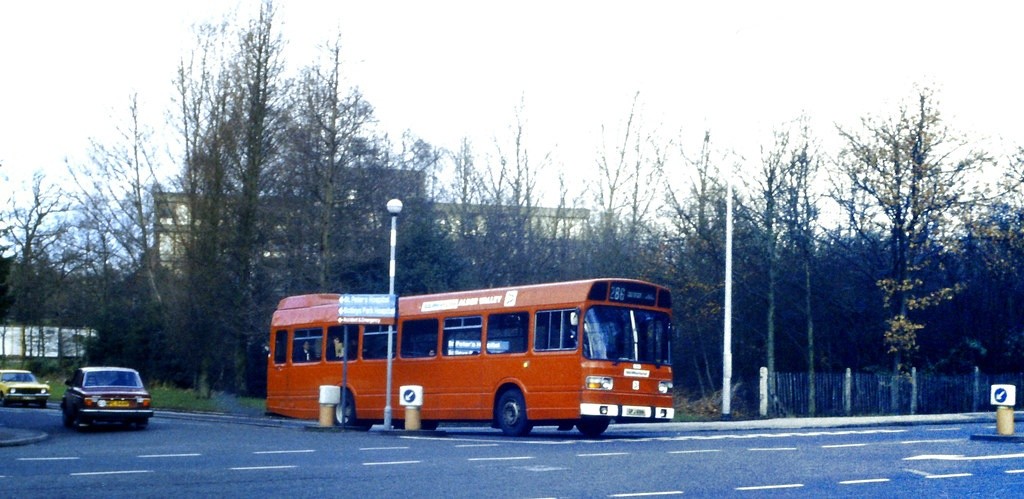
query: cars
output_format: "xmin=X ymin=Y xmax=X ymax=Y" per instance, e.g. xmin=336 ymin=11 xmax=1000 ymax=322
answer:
xmin=61 ymin=366 xmax=153 ymax=430
xmin=0 ymin=369 xmax=52 ymax=407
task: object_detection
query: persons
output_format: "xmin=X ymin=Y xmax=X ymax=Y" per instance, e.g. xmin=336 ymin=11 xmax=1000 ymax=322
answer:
xmin=293 ymin=341 xmax=314 ymax=364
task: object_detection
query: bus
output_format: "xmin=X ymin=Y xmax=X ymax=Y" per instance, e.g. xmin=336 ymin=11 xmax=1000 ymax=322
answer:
xmin=266 ymin=278 xmax=675 ymax=438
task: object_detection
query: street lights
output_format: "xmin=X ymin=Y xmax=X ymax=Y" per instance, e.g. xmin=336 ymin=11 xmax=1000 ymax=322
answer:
xmin=384 ymin=198 xmax=403 ymax=429
